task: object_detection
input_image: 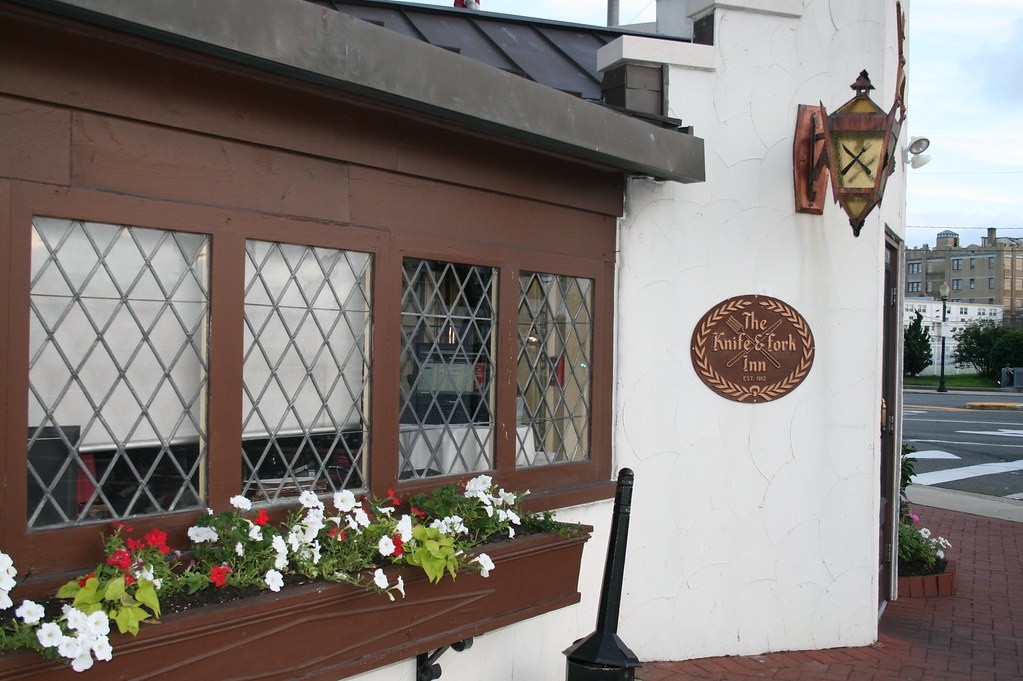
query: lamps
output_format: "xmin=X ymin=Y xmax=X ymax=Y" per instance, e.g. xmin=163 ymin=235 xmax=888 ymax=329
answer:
xmin=793 ymin=69 xmax=907 ymax=238
xmin=902 ymin=135 xmax=933 ymax=169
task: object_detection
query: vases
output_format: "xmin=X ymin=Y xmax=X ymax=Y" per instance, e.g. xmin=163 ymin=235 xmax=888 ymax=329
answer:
xmin=0 ymin=521 xmax=593 ymax=681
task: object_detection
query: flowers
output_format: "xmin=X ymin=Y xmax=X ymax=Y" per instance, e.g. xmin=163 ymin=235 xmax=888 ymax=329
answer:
xmin=0 ymin=474 xmax=579 ymax=673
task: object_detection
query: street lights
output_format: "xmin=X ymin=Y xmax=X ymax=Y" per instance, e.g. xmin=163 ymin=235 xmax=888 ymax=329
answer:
xmin=937 ymin=281 xmax=949 ymax=392
